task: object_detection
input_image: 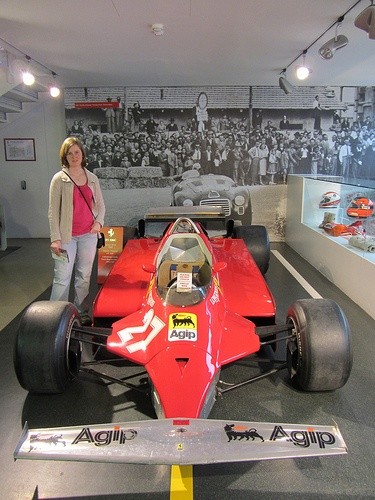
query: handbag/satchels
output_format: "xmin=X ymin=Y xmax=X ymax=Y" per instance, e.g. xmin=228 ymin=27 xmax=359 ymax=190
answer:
xmin=97 ymin=232 xmax=105 ymax=248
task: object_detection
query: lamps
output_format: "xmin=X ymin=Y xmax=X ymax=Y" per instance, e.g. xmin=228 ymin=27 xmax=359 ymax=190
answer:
xmin=354 ymin=0 xmax=374 ymax=39
xmin=278 ymin=70 xmax=294 ymax=93
xmin=21 ymin=54 xmax=35 ymax=86
xmin=319 ymin=21 xmax=352 ymax=58
xmin=49 ymin=73 xmax=61 ymax=97
xmin=296 ymin=50 xmax=311 ymax=80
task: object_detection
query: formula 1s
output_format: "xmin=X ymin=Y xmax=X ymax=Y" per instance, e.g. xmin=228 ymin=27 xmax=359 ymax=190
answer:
xmin=11 ymin=206 xmax=355 ymax=500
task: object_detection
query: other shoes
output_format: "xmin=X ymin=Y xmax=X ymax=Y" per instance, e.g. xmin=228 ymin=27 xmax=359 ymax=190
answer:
xmin=79 ymin=313 xmax=92 ymax=325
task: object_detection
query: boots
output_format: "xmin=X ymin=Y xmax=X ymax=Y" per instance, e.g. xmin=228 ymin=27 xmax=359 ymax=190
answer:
xmin=318 ymin=211 xmax=336 ymax=228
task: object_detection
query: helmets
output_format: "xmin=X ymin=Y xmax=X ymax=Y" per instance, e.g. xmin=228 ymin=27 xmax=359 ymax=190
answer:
xmin=347 ymin=197 xmax=374 ymax=218
xmin=319 ymin=192 xmax=341 ymax=208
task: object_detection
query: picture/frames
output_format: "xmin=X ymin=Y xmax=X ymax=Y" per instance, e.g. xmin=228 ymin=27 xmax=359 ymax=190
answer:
xmin=4 ymin=138 xmax=37 ymax=162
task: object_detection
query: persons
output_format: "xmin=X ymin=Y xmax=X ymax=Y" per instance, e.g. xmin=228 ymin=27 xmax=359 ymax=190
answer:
xmin=68 ymin=96 xmax=375 ymax=187
xmin=49 ymin=137 xmax=104 ymax=326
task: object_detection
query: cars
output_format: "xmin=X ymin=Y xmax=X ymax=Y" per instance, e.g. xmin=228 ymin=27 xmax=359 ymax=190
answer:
xmin=169 ymin=168 xmax=255 ymax=226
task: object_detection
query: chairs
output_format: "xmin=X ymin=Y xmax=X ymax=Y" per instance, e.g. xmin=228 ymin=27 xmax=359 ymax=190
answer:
xmin=155 ymin=233 xmax=212 ymax=286
